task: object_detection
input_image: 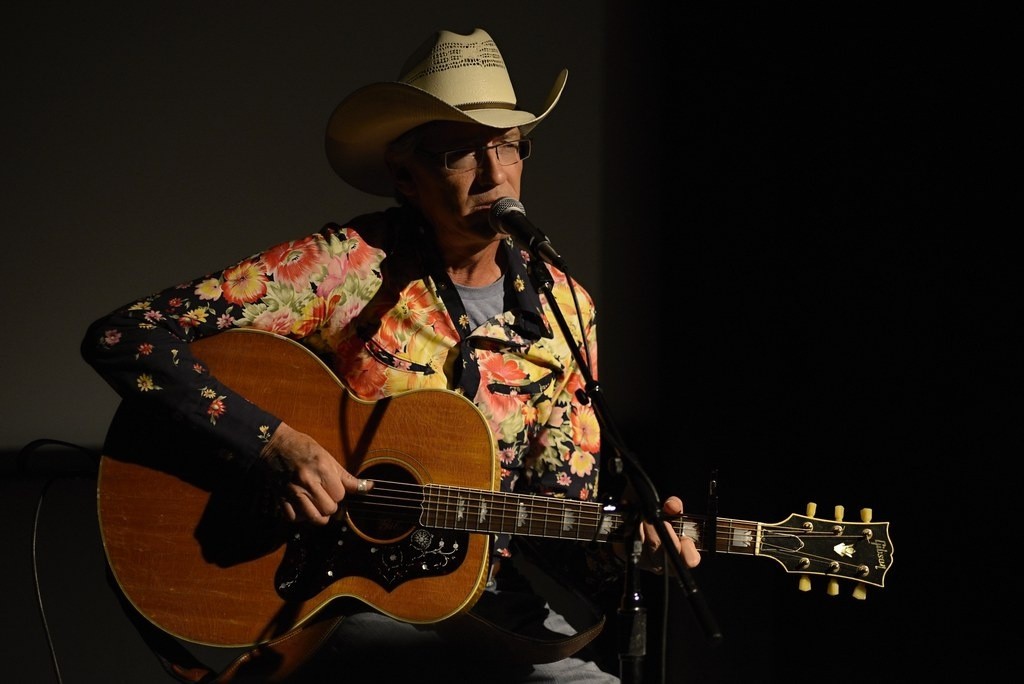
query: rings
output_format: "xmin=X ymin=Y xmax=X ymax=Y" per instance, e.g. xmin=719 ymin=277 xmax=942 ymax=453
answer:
xmin=357 ymin=479 xmax=368 ymax=491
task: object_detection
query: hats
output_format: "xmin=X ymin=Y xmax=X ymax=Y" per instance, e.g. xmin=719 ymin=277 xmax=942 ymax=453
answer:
xmin=322 ymin=27 xmax=569 ymax=196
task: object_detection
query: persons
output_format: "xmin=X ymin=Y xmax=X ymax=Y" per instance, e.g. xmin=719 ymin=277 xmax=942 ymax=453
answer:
xmin=82 ymin=28 xmax=702 ymax=684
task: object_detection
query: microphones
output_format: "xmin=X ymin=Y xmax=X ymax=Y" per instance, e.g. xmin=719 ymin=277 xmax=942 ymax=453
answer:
xmin=487 ymin=195 xmax=568 ymax=275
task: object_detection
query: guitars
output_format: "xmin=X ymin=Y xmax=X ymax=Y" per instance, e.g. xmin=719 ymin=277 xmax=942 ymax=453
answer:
xmin=98 ymin=328 xmax=896 ymax=651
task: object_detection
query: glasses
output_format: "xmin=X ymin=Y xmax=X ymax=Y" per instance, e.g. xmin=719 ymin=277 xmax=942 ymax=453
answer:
xmin=411 ymin=138 xmax=531 ymax=171
